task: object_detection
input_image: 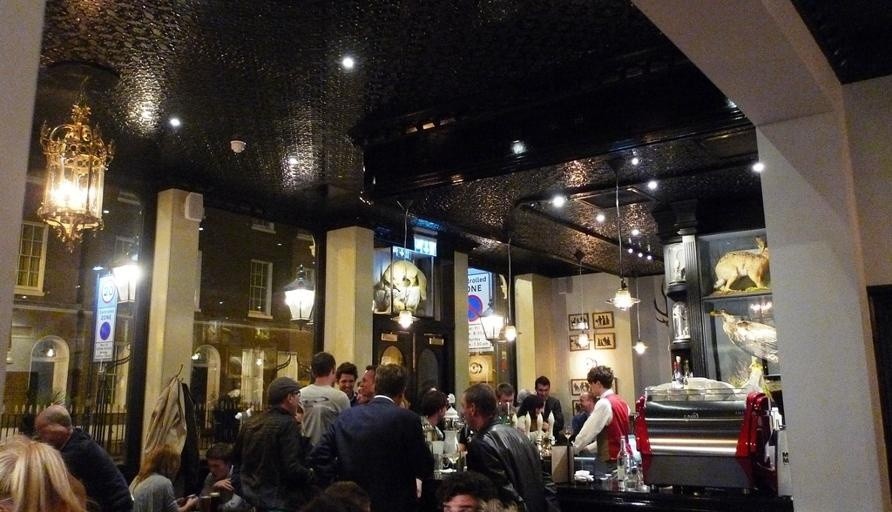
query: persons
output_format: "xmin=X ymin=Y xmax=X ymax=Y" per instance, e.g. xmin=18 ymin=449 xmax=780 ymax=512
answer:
xmin=570 ymin=365 xmax=633 ymax=464
xmin=572 ymin=392 xmax=595 ymax=439
xmin=0 ymin=434 xmax=89 ymax=512
xmin=232 ymin=352 xmax=447 ymax=511
xmin=128 ymin=444 xmax=201 ymax=511
xmin=34 ymin=404 xmax=135 ymax=512
xmin=443 ymin=376 xmax=564 ymax=510
xmin=201 ymin=442 xmax=257 ymax=511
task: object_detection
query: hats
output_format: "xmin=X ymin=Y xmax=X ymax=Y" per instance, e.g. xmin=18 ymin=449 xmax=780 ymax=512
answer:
xmin=268 ymin=376 xmax=306 ymax=402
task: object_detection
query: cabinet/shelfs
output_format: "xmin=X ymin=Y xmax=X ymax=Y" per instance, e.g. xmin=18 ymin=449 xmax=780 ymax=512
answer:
xmin=667 ymin=282 xmax=691 ymax=381
xmin=696 ymin=228 xmax=784 ymax=393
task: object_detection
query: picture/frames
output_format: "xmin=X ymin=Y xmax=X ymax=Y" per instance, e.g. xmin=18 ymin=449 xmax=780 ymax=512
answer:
xmin=568 ymin=312 xmax=589 ymax=332
xmin=594 ymin=377 xmax=617 ymax=400
xmin=592 ymin=311 xmax=614 ymax=329
xmin=593 ymin=332 xmax=615 ymax=350
xmin=572 ymin=399 xmax=582 ymax=416
xmin=571 ymin=378 xmax=592 ymax=396
xmin=569 ymin=334 xmax=590 ymax=351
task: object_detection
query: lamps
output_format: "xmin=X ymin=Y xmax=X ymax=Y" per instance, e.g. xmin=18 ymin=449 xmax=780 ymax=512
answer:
xmin=632 ymin=267 xmax=650 ymax=355
xmin=479 ymin=300 xmax=507 ymax=347
xmin=602 ymin=156 xmax=640 ymax=313
xmin=625 ymin=244 xmax=653 ymax=261
xmin=110 ymin=254 xmax=144 ymax=314
xmin=36 ymin=77 xmax=115 ymax=251
xmin=282 ymin=263 xmax=317 ymax=330
xmin=499 ymin=230 xmax=523 ymax=343
xmin=575 ymin=251 xmax=591 ymax=347
xmin=390 ymin=195 xmax=419 ymax=329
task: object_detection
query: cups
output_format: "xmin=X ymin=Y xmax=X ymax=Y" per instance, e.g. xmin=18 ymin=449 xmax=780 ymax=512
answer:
xmin=181 ymin=492 xmax=224 ymax=512
xmin=426 ymin=441 xmax=444 ymax=473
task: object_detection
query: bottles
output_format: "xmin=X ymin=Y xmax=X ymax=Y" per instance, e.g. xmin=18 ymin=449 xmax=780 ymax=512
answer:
xmin=501 ymin=402 xmax=514 ymax=427
xmin=617 ymin=435 xmax=630 ymax=488
xmin=672 ymin=353 xmax=690 ymax=381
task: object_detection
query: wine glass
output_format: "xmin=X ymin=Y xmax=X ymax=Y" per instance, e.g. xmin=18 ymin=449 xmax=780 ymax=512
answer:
xmin=563 ymin=425 xmax=573 ymax=446
